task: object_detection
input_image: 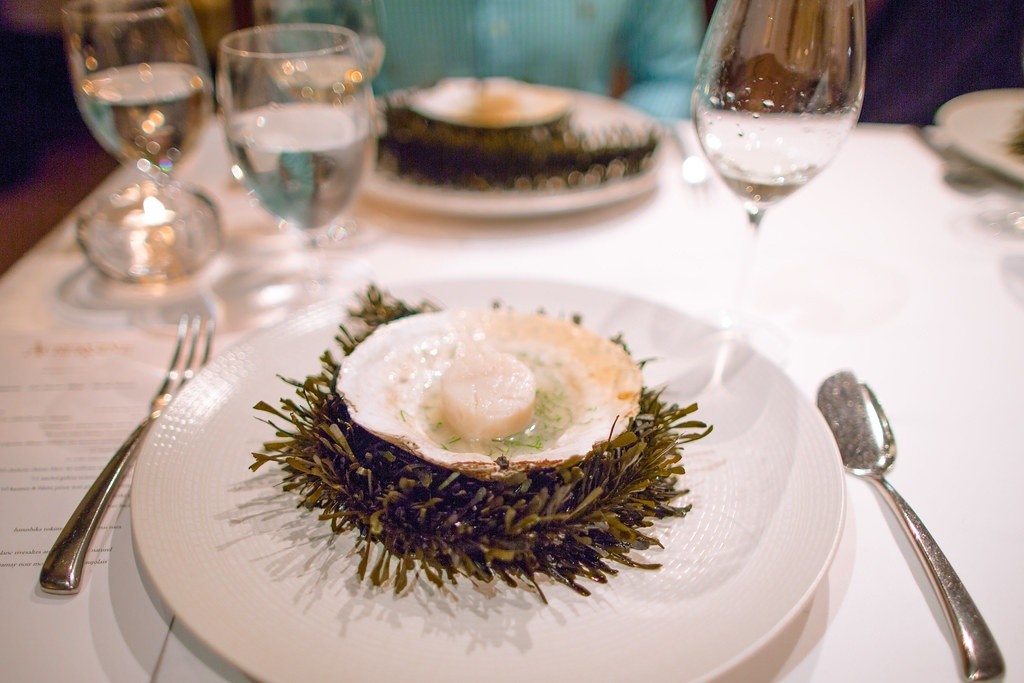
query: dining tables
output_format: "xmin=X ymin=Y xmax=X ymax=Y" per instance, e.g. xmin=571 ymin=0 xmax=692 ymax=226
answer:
xmin=0 ymin=124 xmax=1024 ymax=683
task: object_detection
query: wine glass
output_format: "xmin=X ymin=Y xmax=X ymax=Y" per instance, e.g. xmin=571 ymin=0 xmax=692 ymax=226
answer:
xmin=682 ymin=0 xmax=868 ymax=330
xmin=215 ymin=23 xmax=388 ymax=318
xmin=55 ymin=0 xmax=224 ymax=285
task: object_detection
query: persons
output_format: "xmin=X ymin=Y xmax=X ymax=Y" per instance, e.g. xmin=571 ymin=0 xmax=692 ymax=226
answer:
xmin=274 ymin=0 xmax=707 ymax=119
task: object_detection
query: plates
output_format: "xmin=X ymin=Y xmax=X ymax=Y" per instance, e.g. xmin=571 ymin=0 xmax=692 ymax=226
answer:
xmin=935 ymin=87 xmax=1024 ymax=194
xmin=130 ymin=274 xmax=848 ymax=683
xmin=339 ymin=85 xmax=675 ymax=221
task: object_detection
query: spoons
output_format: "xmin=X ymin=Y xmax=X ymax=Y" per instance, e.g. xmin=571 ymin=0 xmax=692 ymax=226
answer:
xmin=814 ymin=366 xmax=1007 ymax=683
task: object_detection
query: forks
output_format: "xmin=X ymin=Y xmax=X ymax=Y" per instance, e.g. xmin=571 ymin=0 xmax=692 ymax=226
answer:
xmin=37 ymin=311 xmax=218 ymax=595
